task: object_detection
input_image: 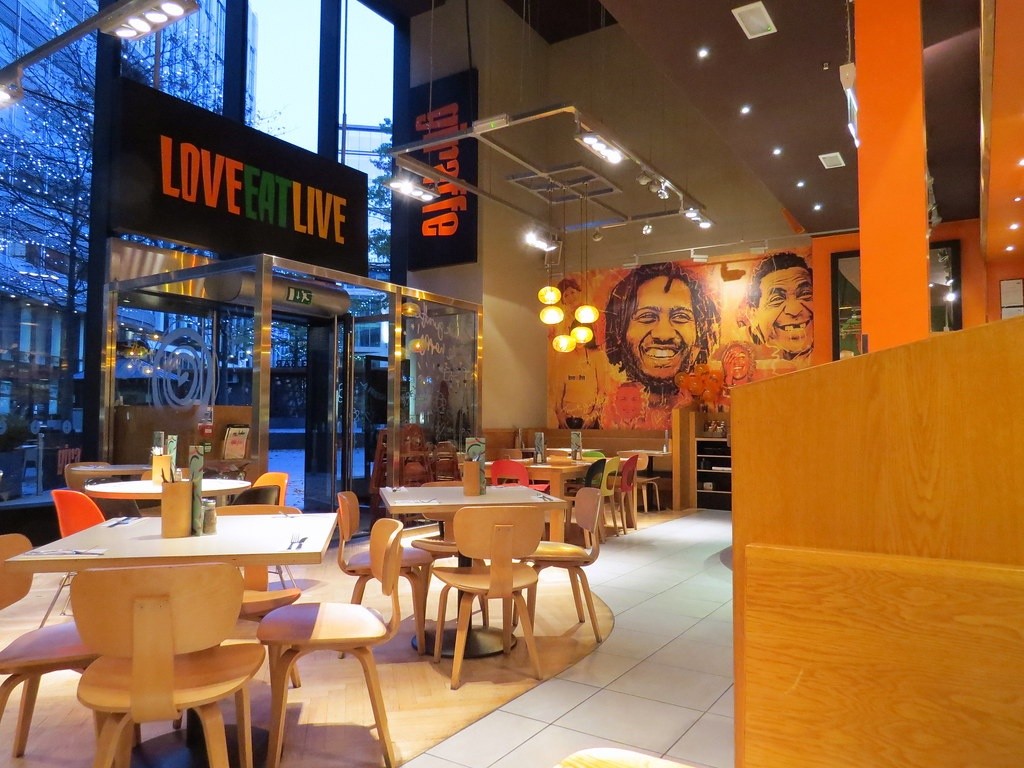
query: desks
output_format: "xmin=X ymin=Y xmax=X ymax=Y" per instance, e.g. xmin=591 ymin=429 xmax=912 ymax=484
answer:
xmin=4 ymin=512 xmax=339 ymax=768
xmin=379 ymin=485 xmax=572 ymax=659
xmin=84 ymin=479 xmax=252 ymax=517
xmin=515 ymin=448 xmax=603 ymax=457
xmin=617 ymin=449 xmax=673 ymax=512
xmin=478 ymin=455 xmax=592 ymax=543
xmin=72 ymin=464 xmax=153 ymax=517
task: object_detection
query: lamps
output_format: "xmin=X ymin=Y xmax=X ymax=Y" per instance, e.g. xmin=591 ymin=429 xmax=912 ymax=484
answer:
xmin=636 ymin=171 xmax=669 ymax=199
xmin=536 ymin=177 xmax=652 ymax=354
xmin=937 ymin=252 xmax=955 ymax=302
xmin=679 ymin=195 xmax=711 ymax=229
xmin=99 ymin=0 xmax=198 ymax=41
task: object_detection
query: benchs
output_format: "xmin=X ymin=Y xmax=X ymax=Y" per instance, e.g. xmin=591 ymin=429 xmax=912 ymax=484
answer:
xmin=528 ymin=428 xmax=673 ymax=492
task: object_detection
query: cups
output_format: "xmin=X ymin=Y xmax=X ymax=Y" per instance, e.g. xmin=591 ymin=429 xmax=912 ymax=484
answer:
xmin=515 ymin=435 xmax=521 ymax=448
xmin=461 ymin=460 xmax=481 ymax=496
xmin=161 ymin=481 xmax=193 ymax=537
xmin=152 ymin=455 xmax=172 ymax=485
xmin=703 ymin=482 xmax=713 ymax=489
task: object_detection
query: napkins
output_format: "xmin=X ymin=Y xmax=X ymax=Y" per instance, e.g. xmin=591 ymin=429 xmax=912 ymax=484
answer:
xmin=103 ymin=518 xmax=147 ymax=526
xmin=25 ymin=546 xmax=109 ymax=557
xmin=395 ymin=499 xmax=438 ymax=505
xmin=386 ymin=486 xmax=407 ymax=492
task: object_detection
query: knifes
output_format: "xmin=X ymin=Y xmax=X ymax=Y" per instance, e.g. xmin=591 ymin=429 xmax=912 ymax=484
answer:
xmin=296 ymin=538 xmax=307 ymax=549
xmin=543 ymin=495 xmax=553 ymax=501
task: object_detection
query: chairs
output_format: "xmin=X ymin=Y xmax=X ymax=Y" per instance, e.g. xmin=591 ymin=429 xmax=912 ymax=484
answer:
xmin=0 ymin=423 xmax=690 ymax=768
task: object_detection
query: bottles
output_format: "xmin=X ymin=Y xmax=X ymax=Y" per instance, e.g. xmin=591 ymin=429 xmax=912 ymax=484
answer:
xmin=702 ymin=458 xmax=706 ymax=468
xmin=570 ymin=448 xmax=581 ymax=460
xmin=175 ymin=468 xmax=182 ymax=482
xmin=199 ymin=497 xmax=216 ymax=534
xmin=662 ymin=442 xmax=667 ymax=454
xmin=521 ymin=441 xmax=525 ymax=448
xmin=533 ymin=450 xmax=541 ymax=464
xmin=704 ymin=419 xmax=726 ymax=431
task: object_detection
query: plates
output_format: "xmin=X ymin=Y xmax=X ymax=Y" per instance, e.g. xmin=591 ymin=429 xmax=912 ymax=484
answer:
xmin=704 ymin=447 xmax=723 ymax=450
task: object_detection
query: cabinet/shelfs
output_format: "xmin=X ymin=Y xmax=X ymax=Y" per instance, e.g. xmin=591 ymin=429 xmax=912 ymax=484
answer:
xmin=696 ymin=433 xmax=732 ymax=513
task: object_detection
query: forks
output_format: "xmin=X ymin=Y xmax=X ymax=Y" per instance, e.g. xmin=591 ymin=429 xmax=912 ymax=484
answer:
xmin=536 ymin=492 xmax=546 ymax=501
xmin=287 ymin=533 xmax=299 ymax=550
